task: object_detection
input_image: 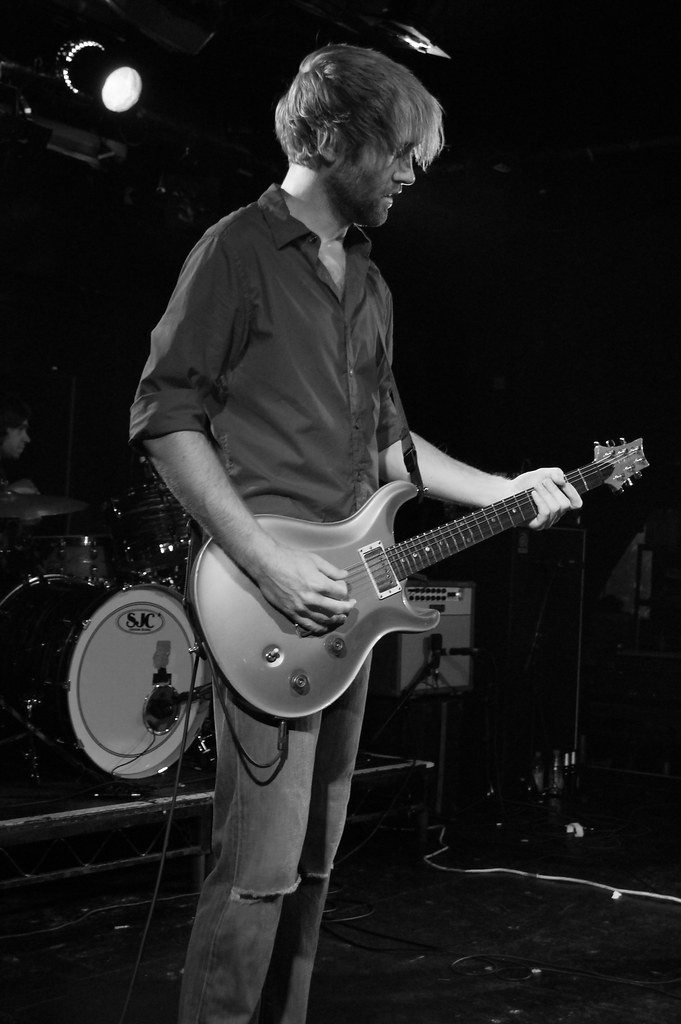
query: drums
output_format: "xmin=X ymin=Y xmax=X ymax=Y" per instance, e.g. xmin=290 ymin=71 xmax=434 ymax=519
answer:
xmin=100 ymin=477 xmax=194 ymax=575
xmin=1 ymin=534 xmax=114 ymax=585
xmin=1 ymin=573 xmax=209 ymax=782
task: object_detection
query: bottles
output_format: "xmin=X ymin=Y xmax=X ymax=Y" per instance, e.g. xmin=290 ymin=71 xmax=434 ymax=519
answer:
xmin=549 ymin=751 xmax=566 ymax=795
xmin=529 ymin=751 xmax=544 ymax=792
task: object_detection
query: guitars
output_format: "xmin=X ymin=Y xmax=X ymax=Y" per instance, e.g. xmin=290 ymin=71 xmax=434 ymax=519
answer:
xmin=183 ymin=435 xmax=652 ymax=727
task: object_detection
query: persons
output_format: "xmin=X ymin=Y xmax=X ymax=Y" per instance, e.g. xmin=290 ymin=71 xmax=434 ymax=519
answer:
xmin=130 ymin=45 xmax=586 ymax=1024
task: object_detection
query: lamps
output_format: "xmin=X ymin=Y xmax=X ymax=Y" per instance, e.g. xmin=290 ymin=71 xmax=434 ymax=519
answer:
xmin=53 ymin=40 xmax=142 ymax=113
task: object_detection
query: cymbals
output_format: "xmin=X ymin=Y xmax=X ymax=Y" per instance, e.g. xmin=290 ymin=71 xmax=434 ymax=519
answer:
xmin=0 ymin=494 xmax=89 ymax=520
